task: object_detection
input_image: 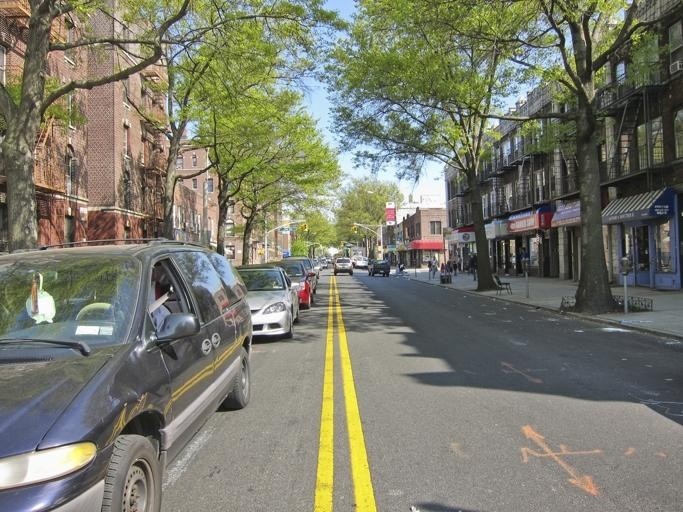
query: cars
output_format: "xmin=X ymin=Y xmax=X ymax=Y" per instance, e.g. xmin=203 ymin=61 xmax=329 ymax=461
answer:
xmin=333 ymin=255 xmax=390 ymax=277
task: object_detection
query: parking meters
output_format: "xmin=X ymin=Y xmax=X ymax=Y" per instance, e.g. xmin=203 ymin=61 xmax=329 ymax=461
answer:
xmin=619 ymin=257 xmax=630 ymax=315
xmin=521 ymin=256 xmax=530 ymax=298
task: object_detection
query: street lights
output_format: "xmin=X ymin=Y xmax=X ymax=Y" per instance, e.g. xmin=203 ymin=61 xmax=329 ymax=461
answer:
xmin=264 ymin=219 xmax=306 ymax=263
xmin=366 ymin=190 xmax=399 ymax=275
xmin=354 ymin=223 xmax=383 ymax=261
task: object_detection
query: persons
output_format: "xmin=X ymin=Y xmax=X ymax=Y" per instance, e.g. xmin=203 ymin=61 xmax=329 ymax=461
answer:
xmin=431 ymin=255 xmax=462 ymax=280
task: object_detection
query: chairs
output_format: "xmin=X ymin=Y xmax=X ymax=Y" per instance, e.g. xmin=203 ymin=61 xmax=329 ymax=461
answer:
xmin=494 ymin=275 xmax=512 ymax=296
